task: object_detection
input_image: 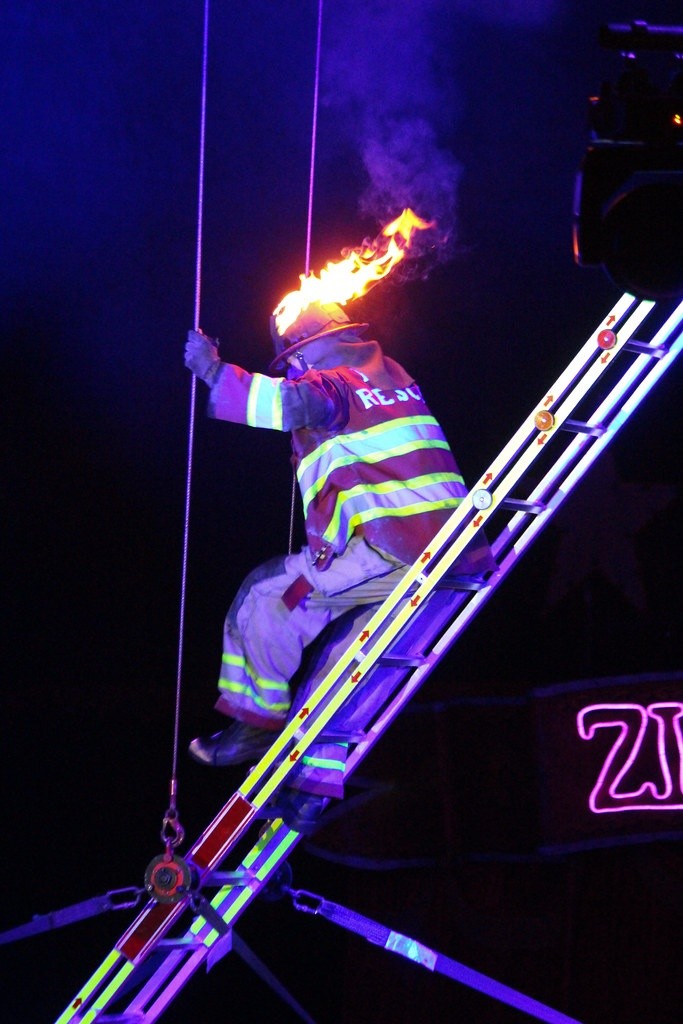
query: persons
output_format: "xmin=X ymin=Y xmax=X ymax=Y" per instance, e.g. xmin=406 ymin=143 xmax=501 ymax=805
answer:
xmin=184 ymin=290 xmax=502 ymax=832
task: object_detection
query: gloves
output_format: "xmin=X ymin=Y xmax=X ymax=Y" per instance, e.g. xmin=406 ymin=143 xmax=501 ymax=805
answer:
xmin=184 ymin=327 xmax=220 ymax=389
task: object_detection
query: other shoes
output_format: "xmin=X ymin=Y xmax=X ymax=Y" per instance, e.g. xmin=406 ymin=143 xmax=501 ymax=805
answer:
xmin=257 ymin=786 xmax=329 ymax=833
xmin=187 ymin=715 xmax=282 ymax=769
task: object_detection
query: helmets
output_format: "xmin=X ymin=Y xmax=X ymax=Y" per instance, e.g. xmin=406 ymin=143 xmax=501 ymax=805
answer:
xmin=268 ymin=291 xmax=369 ymax=377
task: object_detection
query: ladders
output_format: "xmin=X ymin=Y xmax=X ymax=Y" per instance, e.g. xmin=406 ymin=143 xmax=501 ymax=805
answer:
xmin=57 ymin=292 xmax=682 ymax=1024
